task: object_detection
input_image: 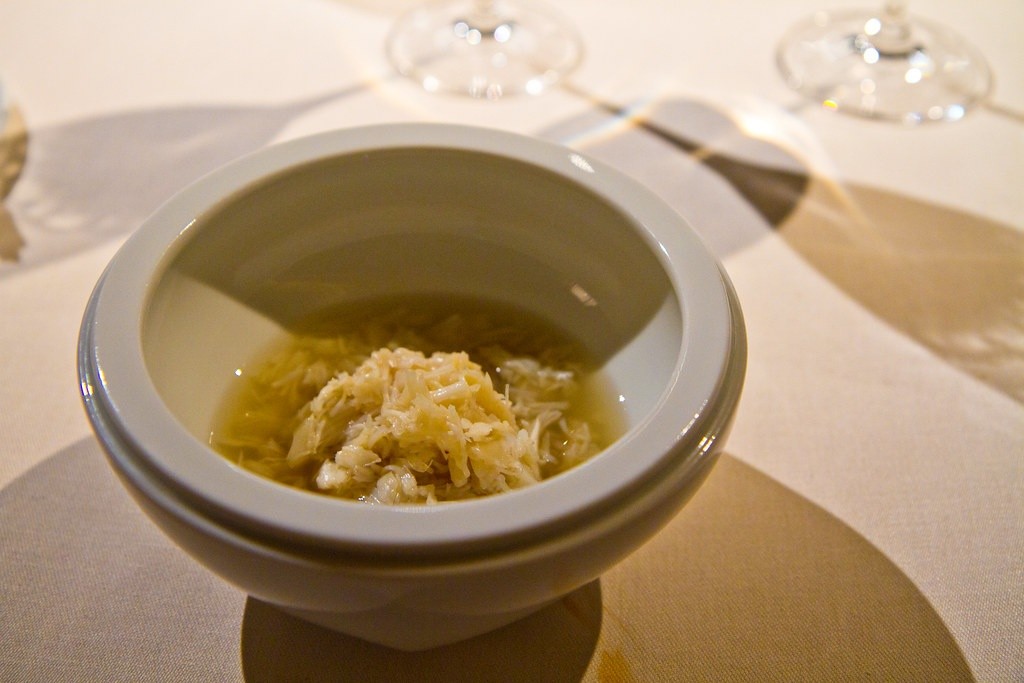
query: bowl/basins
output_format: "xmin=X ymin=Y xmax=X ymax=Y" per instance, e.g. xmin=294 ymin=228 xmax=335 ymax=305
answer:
xmin=78 ymin=123 xmax=745 ymax=651
